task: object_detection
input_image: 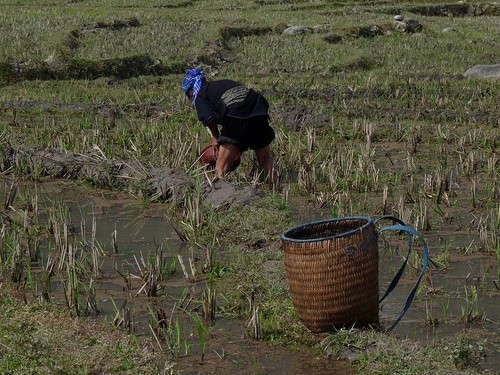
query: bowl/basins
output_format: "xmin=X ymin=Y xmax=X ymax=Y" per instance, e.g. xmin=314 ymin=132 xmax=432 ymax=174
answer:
xmin=200 ymin=142 xmax=241 ymax=170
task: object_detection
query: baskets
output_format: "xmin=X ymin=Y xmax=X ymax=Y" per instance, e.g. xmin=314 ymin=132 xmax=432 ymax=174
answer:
xmin=281 ymin=215 xmax=430 ymax=334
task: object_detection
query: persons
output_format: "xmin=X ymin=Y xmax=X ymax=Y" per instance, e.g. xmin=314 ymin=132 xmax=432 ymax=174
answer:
xmin=181 ymin=67 xmax=278 ymax=183
